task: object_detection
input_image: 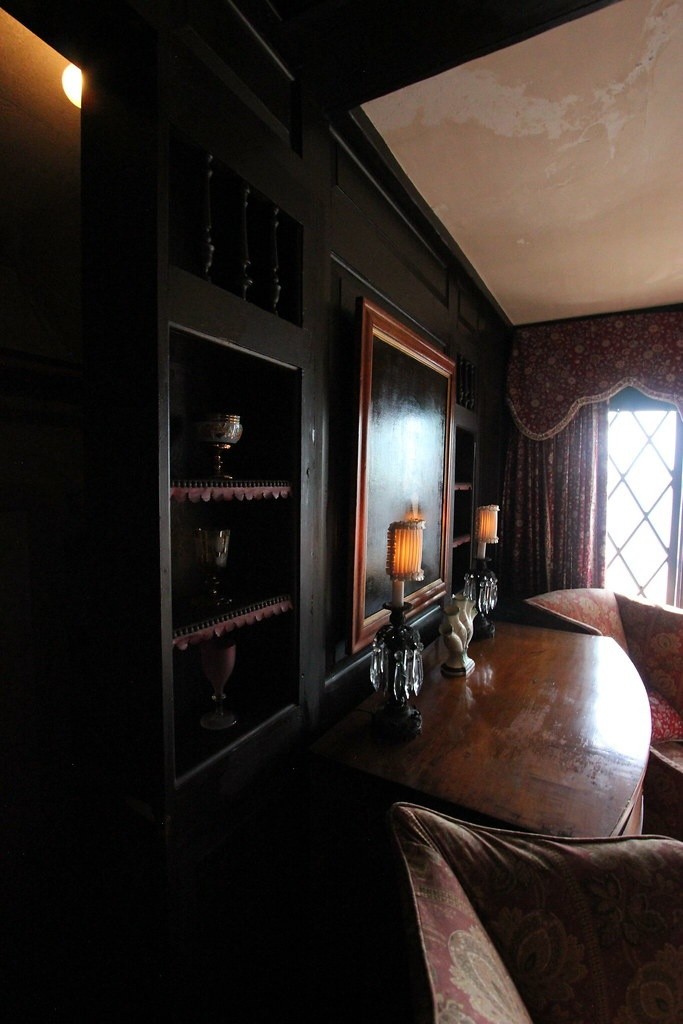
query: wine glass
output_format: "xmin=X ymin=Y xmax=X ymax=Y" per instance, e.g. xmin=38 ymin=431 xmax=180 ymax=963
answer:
xmin=196 ymin=527 xmax=233 ymax=606
xmin=198 ymin=639 xmax=238 ymax=728
xmin=193 ymin=413 xmax=244 ymax=482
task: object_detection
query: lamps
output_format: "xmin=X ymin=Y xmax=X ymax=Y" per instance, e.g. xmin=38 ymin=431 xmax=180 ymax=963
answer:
xmin=462 ymin=505 xmax=502 ymax=641
xmin=368 ymin=519 xmax=425 ymax=731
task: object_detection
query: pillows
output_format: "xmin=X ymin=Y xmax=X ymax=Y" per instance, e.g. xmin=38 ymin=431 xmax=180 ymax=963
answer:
xmin=646 ymin=688 xmax=683 ymax=745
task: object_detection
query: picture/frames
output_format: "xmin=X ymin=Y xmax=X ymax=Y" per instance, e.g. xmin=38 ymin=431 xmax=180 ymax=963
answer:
xmin=352 ymin=298 xmax=450 ymax=655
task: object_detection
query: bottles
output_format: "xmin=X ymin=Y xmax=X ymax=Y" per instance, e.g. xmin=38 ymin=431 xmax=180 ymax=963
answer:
xmin=180 ymin=149 xmax=285 ymax=316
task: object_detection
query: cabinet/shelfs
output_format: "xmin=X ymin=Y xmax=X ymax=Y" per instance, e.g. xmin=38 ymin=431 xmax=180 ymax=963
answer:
xmin=80 ymin=76 xmax=323 ymax=906
xmin=347 ymin=619 xmax=654 ymax=837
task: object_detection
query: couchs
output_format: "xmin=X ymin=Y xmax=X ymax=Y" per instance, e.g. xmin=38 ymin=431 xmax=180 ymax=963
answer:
xmin=523 ymin=585 xmax=683 ymax=794
xmin=383 ymin=801 xmax=683 ymax=1024
